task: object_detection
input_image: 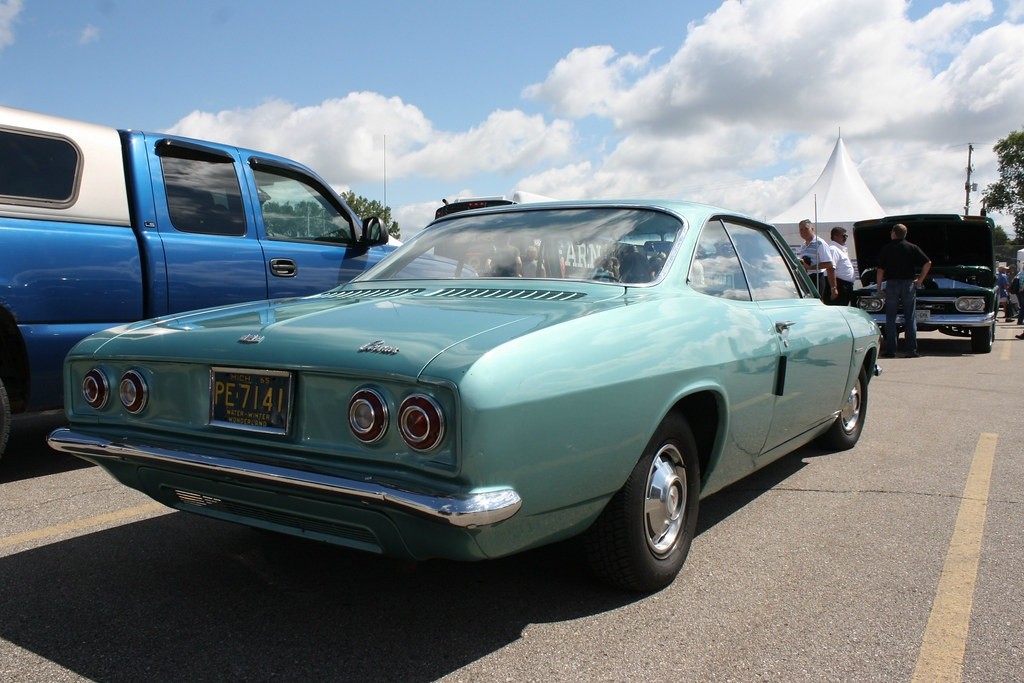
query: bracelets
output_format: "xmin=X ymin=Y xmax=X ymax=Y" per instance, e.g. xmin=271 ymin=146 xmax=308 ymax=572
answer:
xmin=831 ymin=286 xmax=837 ymax=291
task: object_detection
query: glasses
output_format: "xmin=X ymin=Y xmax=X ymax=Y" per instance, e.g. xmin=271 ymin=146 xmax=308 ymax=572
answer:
xmin=837 ymin=235 xmax=848 ymax=238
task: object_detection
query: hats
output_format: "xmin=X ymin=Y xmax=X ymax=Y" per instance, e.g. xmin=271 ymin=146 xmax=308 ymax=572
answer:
xmin=998 ymin=266 xmax=1009 ymax=272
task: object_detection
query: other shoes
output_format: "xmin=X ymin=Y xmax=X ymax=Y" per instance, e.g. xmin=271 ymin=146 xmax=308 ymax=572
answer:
xmin=907 ymin=352 xmax=919 ymax=357
xmin=881 ymin=354 xmax=895 ymax=358
xmin=1015 ymin=334 xmax=1024 ymax=340
xmin=1005 ymin=319 xmax=1014 ymax=323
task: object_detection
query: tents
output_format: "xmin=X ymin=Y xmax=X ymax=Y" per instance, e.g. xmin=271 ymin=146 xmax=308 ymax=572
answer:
xmin=768 ymin=136 xmax=890 ymax=258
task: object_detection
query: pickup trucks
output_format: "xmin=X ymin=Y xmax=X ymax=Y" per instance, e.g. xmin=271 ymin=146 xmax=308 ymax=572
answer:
xmin=0 ymin=104 xmax=480 ymax=457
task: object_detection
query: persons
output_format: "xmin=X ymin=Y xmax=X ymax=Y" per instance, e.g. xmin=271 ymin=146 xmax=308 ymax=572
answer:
xmin=478 ymin=234 xmax=666 ymax=285
xmin=799 ymin=219 xmax=932 ymax=358
xmin=997 ymin=262 xmax=1024 ymax=340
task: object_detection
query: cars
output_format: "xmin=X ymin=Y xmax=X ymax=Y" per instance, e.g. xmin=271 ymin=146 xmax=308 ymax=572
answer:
xmin=850 ymin=213 xmax=1005 ymax=352
xmin=61 ymin=193 xmax=883 ymax=591
